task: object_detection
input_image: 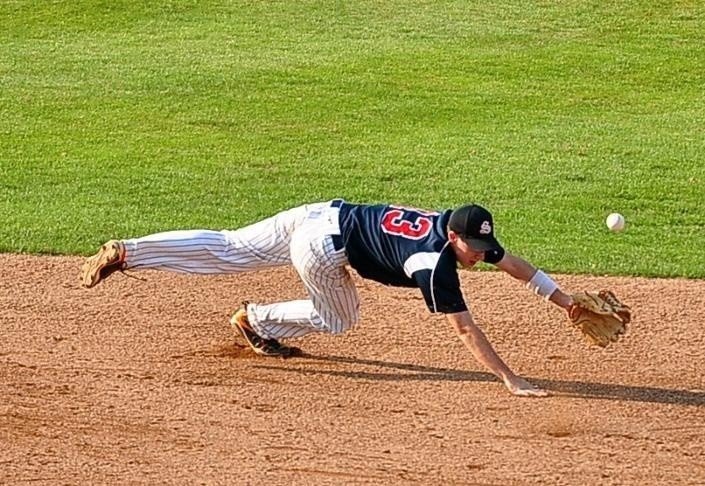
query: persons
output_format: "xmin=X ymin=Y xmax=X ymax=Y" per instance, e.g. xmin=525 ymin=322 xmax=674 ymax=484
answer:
xmin=77 ymin=196 xmax=632 ymax=399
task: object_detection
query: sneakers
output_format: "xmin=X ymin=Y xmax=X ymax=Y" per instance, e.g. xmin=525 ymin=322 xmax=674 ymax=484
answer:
xmin=77 ymin=238 xmax=125 ymax=290
xmin=230 ymin=301 xmax=288 ymax=355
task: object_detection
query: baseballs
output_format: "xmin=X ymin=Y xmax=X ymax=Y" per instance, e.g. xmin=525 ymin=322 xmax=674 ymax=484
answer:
xmin=606 ymin=213 xmax=625 ymax=230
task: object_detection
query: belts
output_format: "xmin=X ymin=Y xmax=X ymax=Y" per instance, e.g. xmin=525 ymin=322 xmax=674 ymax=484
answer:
xmin=329 ymin=199 xmax=345 ymax=253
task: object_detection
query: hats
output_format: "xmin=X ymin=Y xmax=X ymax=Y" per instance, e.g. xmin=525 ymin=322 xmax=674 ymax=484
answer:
xmin=448 ymin=204 xmax=499 ymax=251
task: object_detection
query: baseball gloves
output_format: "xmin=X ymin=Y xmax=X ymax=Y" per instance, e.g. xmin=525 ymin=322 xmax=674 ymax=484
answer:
xmin=569 ymin=290 xmax=632 ymax=348
